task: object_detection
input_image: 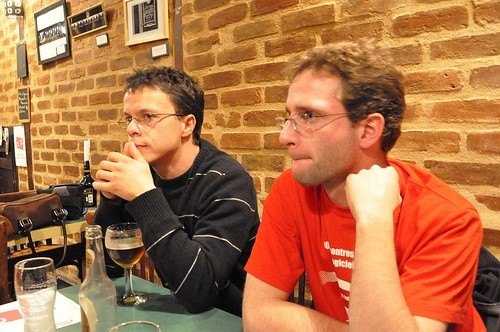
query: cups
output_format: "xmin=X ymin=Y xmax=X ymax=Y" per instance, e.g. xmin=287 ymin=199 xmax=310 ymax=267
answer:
xmin=13 ymin=257 xmax=58 ymax=332
xmin=110 ymin=321 xmax=161 ymax=332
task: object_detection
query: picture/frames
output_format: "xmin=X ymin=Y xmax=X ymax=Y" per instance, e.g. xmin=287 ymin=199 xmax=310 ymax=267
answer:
xmin=66 ymin=2 xmax=108 ymax=38
xmin=123 ymin=0 xmax=170 ymax=46
xmin=34 ymin=0 xmax=71 ymax=65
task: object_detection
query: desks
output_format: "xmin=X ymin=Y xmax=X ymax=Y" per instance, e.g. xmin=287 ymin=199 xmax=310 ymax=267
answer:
xmin=6 ymin=205 xmax=98 ymax=283
xmin=54 ymin=275 xmax=245 ymax=332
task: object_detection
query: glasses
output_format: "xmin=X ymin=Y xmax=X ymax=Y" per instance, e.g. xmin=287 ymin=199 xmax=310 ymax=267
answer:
xmin=118 ymin=113 xmax=186 ymax=129
xmin=275 ymin=110 xmax=373 ymax=132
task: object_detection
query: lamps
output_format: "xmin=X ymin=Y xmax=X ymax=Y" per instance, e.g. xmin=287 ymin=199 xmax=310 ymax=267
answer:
xmin=2 ymin=1 xmax=24 ymax=19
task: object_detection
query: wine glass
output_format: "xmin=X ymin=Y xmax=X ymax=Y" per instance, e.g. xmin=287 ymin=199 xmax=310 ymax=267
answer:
xmin=104 ymin=222 xmax=150 ymax=308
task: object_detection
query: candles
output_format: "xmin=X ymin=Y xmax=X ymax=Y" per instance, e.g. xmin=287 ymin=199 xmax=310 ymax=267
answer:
xmin=84 ymin=139 xmax=90 ymax=161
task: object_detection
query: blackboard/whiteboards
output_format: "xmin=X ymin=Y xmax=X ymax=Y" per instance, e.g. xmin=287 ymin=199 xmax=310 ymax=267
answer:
xmin=17 ymin=88 xmax=32 ymax=123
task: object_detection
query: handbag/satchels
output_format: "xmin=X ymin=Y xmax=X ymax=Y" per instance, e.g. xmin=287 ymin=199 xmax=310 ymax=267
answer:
xmin=48 ymin=185 xmax=89 ymax=220
xmin=0 ymin=192 xmax=68 ymax=267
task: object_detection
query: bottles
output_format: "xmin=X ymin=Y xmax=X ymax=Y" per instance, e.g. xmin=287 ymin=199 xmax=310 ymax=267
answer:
xmin=78 ymin=225 xmax=118 ymax=332
xmin=77 ymin=160 xmax=97 ymax=208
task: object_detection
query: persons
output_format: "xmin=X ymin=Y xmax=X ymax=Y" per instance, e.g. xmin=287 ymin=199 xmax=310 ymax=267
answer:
xmin=92 ymin=65 xmax=261 ymax=315
xmin=242 ymin=41 xmax=488 ymax=332
